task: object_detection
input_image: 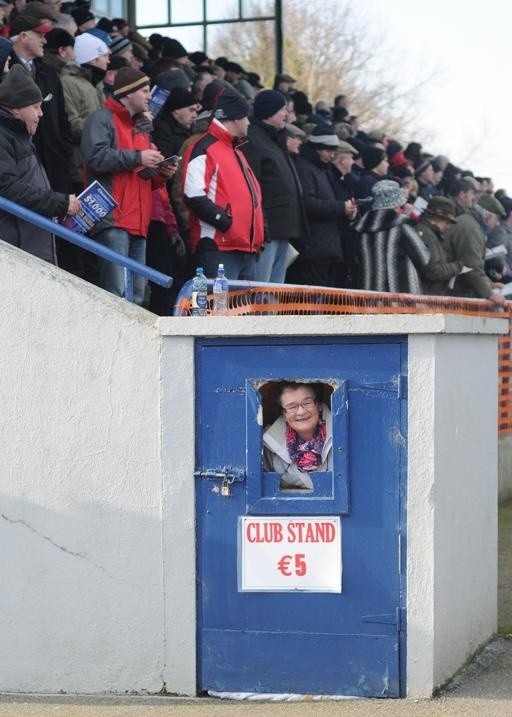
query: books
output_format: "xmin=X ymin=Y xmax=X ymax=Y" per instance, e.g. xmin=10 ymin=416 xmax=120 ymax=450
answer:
xmin=53 ymin=180 xmax=116 ymax=237
xmin=147 ymin=85 xmax=171 ymax=120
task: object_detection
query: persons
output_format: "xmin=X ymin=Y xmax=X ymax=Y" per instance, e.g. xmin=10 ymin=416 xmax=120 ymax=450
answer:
xmin=60 ymin=33 xmax=112 ymax=158
xmin=188 ymin=51 xmax=359 ymax=139
xmin=0 ymin=63 xmax=81 ymax=269
xmin=239 ymin=89 xmax=309 ymax=304
xmin=152 ymin=85 xmax=197 ymax=160
xmin=333 ymin=139 xmax=366 ymax=290
xmin=0 ymin=0 xmax=164 ymax=70
xmin=486 ymin=197 xmax=512 ymax=285
xmin=0 ymin=14 xmax=72 ymax=171
xmin=181 ymin=88 xmax=264 ymax=281
xmin=413 ymin=195 xmax=464 ymax=297
xmin=442 ymin=194 xmax=506 ymax=306
xmin=146 ymin=40 xmax=187 ymax=88
xmin=81 ymin=68 xmax=179 ymax=305
xmin=263 ymin=382 xmax=333 ymax=490
xmin=105 ymin=57 xmax=130 ymax=95
xmin=359 ymin=123 xmax=510 ymax=196
xmin=349 ymin=180 xmax=463 ymax=308
xmin=296 ymin=126 xmax=359 ymax=287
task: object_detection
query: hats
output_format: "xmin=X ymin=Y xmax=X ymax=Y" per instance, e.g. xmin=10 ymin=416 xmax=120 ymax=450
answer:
xmin=84 ymin=28 xmax=112 ymax=44
xmin=0 ymin=64 xmax=43 ymax=109
xmin=71 ymin=9 xmax=94 ymax=26
xmin=421 ymin=196 xmax=457 ymax=224
xmin=307 ymin=123 xmax=339 ymax=150
xmin=477 ymin=194 xmax=507 ymax=218
xmin=43 ymin=27 xmax=75 ymax=49
xmin=254 ymin=89 xmax=286 ymax=120
xmin=413 ymin=158 xmax=428 ymax=174
xmin=73 ymin=32 xmax=112 ymax=64
xmin=8 ymin=15 xmax=54 ymax=38
xmin=158 ymin=36 xmax=188 ymax=59
xmin=285 ymin=124 xmax=306 ymax=138
xmin=110 ymin=36 xmax=132 ymax=56
xmin=112 ymin=65 xmax=151 ymax=97
xmin=166 ymin=85 xmax=197 ymax=111
xmin=429 ymin=155 xmax=449 ymax=171
xmin=338 ymin=139 xmax=359 ymax=155
xmin=362 ymin=146 xmax=387 ymax=171
xmin=371 ymin=180 xmax=408 ymax=210
xmin=202 ymin=78 xmax=249 ymax=120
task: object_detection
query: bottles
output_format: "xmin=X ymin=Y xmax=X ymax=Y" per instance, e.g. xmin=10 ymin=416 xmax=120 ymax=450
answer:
xmin=213 ymin=263 xmax=228 ymax=311
xmin=191 ymin=267 xmax=207 ymax=316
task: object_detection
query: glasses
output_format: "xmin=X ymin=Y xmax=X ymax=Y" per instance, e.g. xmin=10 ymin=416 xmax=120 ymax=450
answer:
xmin=281 ymin=396 xmax=317 ymax=414
